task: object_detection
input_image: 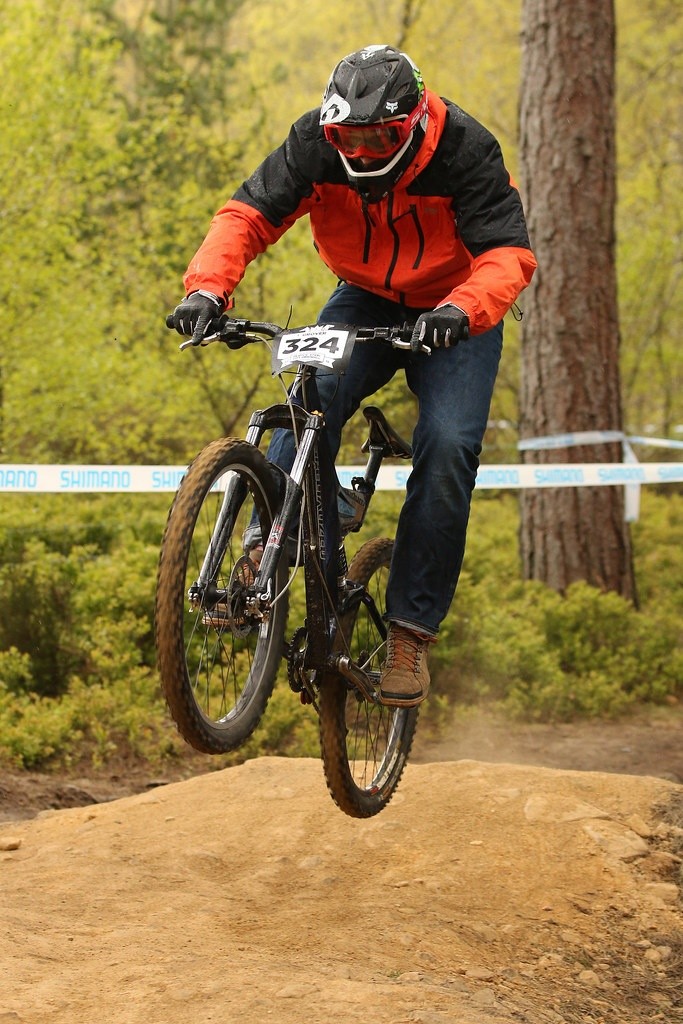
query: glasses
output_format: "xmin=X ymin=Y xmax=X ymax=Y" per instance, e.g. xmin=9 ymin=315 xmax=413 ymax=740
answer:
xmin=325 ymin=121 xmax=404 ymax=161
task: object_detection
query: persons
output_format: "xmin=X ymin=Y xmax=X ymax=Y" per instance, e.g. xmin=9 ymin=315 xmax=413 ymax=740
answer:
xmin=172 ymin=44 xmax=540 ymax=709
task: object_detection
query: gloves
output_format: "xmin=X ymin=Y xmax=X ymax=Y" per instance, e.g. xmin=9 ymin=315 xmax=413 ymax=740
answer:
xmin=410 ymin=301 xmax=469 ymax=352
xmin=173 ymin=290 xmax=221 ymax=347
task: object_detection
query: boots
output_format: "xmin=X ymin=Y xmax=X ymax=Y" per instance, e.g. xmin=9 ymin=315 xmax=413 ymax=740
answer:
xmin=379 ymin=621 xmax=430 ymax=708
xmin=201 ymin=549 xmax=268 ymax=627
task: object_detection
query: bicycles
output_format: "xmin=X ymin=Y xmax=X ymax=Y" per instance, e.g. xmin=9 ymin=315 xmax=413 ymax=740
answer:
xmin=152 ymin=309 xmax=471 ymax=820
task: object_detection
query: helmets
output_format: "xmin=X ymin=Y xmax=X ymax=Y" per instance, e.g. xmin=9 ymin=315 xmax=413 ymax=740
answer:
xmin=320 ymin=45 xmax=429 ymax=204
